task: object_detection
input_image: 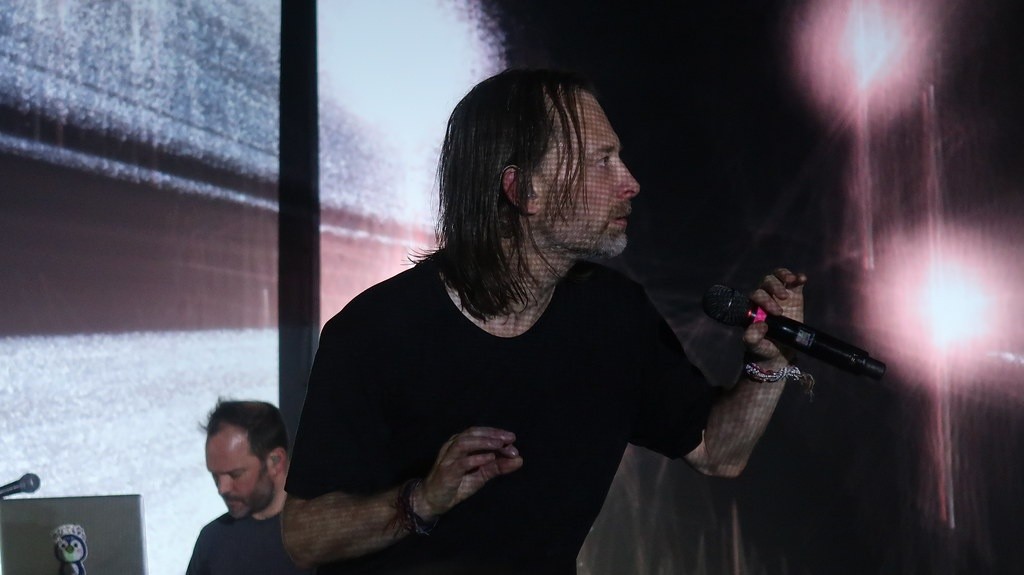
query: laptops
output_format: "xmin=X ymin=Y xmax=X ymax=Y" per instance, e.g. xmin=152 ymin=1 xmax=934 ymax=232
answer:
xmin=1 ymin=493 xmax=148 ymax=575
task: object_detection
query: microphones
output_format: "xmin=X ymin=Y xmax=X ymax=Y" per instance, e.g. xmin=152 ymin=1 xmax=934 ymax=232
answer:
xmin=0 ymin=473 xmax=40 ymax=498
xmin=701 ymin=285 xmax=886 ymax=382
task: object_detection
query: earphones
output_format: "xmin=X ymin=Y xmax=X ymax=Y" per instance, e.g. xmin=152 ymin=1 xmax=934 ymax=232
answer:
xmin=273 ymin=455 xmax=281 ymax=467
xmin=516 ymin=176 xmax=535 ymax=198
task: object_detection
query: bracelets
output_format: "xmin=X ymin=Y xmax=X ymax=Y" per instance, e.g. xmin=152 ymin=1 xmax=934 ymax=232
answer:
xmin=396 ymin=477 xmax=438 ymax=536
xmin=742 ymin=360 xmax=814 ymax=393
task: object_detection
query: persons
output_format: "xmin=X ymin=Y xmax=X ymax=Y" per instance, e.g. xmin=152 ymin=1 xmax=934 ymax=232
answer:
xmin=279 ymin=67 xmax=815 ymax=575
xmin=186 ymin=401 xmax=291 ymax=574
